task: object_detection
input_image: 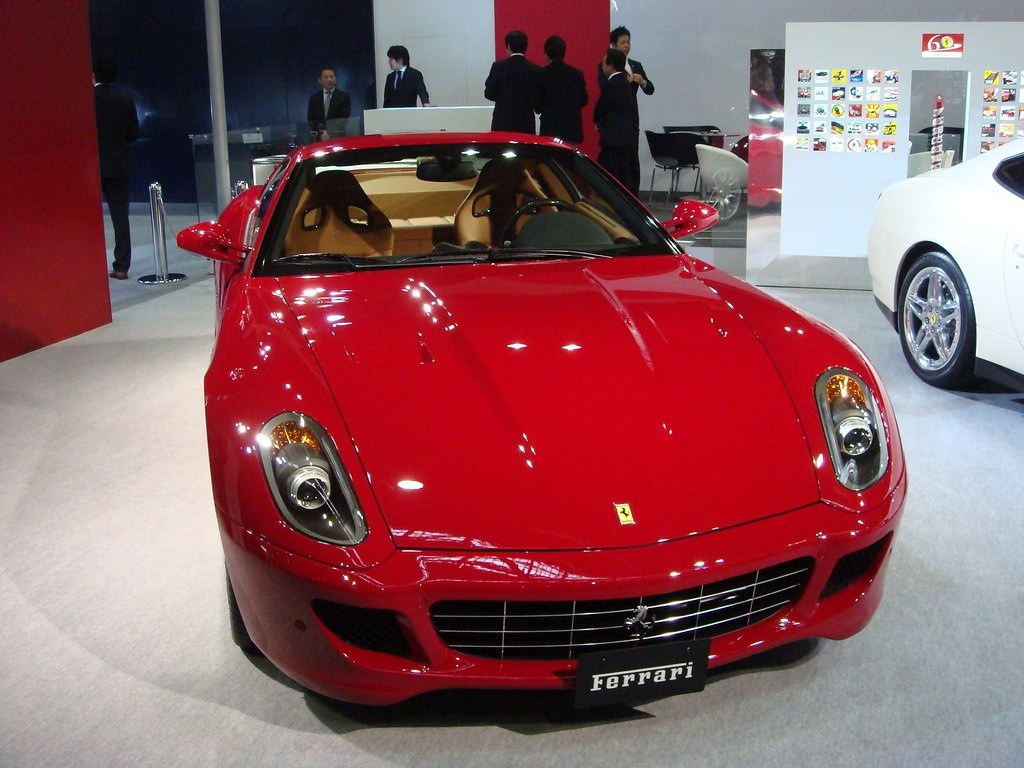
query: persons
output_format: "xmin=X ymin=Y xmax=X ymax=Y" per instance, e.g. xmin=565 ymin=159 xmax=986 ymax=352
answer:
xmin=94 ymin=55 xmax=142 ymax=279
xmin=485 ymin=31 xmax=543 ymax=134
xmin=593 ymin=27 xmax=654 ymax=197
xmin=535 ymin=36 xmax=588 ymax=146
xmin=381 ymin=45 xmax=436 ymax=110
xmin=308 ymin=66 xmax=352 ymax=141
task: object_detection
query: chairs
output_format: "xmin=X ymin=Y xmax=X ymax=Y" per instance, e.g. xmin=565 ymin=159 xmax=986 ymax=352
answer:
xmin=285 ymin=170 xmax=394 ymax=257
xmin=455 ymin=158 xmax=559 ymax=248
xmin=643 ymin=125 xmax=749 ymax=239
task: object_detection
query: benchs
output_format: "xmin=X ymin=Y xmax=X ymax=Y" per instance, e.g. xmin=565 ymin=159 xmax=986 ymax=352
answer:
xmin=348 ymin=171 xmax=491 ymax=257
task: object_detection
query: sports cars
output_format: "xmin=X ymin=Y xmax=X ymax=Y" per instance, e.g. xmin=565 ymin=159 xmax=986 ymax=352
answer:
xmin=176 ymin=126 xmax=908 ymax=716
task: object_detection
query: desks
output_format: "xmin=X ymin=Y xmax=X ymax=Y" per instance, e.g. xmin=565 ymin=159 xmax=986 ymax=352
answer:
xmin=671 ymin=131 xmax=739 ymax=204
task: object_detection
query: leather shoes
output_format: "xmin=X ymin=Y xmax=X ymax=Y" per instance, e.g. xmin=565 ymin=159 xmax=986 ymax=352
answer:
xmin=109 ymin=271 xmax=129 ymax=280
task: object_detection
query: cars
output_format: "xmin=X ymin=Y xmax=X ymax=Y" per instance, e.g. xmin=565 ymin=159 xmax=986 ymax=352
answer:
xmin=747 ymin=88 xmax=784 ymax=212
xmin=867 ymin=135 xmax=1024 ymax=391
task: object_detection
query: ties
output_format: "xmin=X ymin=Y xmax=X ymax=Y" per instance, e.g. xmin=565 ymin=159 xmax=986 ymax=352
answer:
xmin=395 ymin=71 xmax=402 ymax=93
xmin=324 ymin=91 xmax=331 ymax=119
